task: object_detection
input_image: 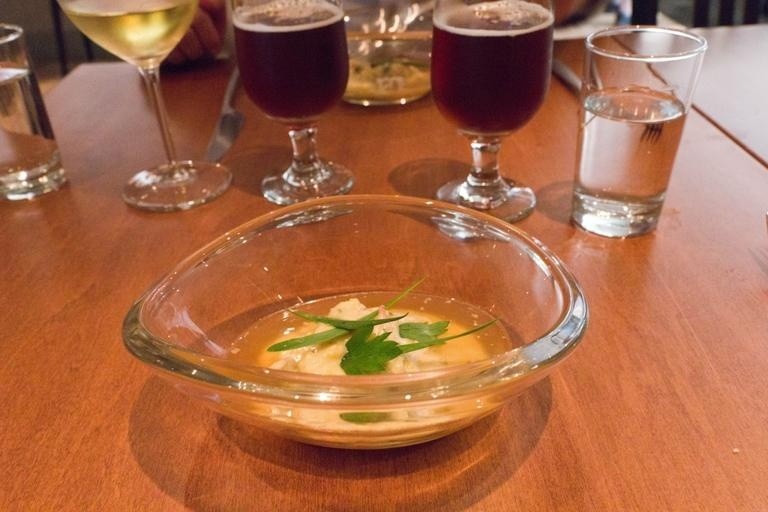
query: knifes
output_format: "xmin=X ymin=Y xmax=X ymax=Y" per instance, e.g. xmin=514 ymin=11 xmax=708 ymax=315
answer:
xmin=205 ymin=57 xmax=244 ymax=176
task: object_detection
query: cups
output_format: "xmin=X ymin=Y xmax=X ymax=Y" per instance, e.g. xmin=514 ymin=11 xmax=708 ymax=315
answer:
xmin=571 ymin=25 xmax=709 ymax=238
xmin=0 ymin=24 xmax=70 ymax=199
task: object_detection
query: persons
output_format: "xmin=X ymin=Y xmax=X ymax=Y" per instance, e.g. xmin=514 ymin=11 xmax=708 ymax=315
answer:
xmin=163 ymin=0 xmax=611 ymax=68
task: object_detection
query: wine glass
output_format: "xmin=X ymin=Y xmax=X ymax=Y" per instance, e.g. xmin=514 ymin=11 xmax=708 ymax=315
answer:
xmin=232 ymin=1 xmax=356 ymax=207
xmin=430 ymin=1 xmax=556 ymax=221
xmin=58 ymin=0 xmax=232 ymax=216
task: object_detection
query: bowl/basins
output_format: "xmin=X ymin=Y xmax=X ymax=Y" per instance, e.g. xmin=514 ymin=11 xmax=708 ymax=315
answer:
xmin=337 ymin=0 xmax=433 ymax=109
xmin=121 ymin=196 xmax=590 ymax=453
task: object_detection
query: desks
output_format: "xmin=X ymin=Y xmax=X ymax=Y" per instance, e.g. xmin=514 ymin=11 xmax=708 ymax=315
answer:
xmin=0 ymin=24 xmax=768 ymax=512
